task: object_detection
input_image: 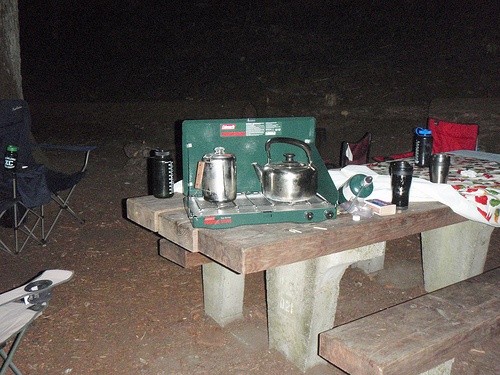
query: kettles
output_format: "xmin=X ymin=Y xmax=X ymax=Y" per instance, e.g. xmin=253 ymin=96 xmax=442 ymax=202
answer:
xmin=251 ymin=137 xmax=318 ymax=204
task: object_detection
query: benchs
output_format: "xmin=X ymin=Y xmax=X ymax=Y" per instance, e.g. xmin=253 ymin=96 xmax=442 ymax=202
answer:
xmin=157 ymin=235 xmax=500 ymax=375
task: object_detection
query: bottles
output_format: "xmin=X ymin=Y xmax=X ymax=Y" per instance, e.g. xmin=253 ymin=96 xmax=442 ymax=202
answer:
xmin=389 ymin=160 xmax=413 ymax=210
xmin=412 ymin=127 xmax=432 ymax=167
xmin=4 ymin=145 xmax=19 ymax=174
xmin=338 ymin=174 xmax=375 ymax=204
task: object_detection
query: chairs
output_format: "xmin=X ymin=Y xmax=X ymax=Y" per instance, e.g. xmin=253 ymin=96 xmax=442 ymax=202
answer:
xmin=0 ymin=96 xmax=95 ymax=256
xmin=0 ymin=267 xmax=76 ymax=375
xmin=316 ymin=116 xmax=479 ymax=169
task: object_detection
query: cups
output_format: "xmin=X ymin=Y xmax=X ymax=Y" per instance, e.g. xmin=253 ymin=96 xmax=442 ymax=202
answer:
xmin=149 ymin=148 xmax=175 ymax=198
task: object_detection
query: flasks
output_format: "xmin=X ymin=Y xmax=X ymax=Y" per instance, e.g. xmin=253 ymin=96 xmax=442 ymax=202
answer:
xmin=428 ymin=153 xmax=450 ymax=183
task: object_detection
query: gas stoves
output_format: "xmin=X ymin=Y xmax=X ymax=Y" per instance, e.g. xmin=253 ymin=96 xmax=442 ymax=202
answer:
xmin=182 ymin=119 xmax=338 ymax=227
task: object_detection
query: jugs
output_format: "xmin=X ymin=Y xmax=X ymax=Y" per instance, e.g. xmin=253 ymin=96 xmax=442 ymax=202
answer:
xmin=195 ymin=146 xmax=237 ymax=203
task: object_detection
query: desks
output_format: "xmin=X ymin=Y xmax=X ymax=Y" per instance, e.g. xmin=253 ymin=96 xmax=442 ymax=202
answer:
xmin=124 ymin=149 xmax=500 ymax=375
